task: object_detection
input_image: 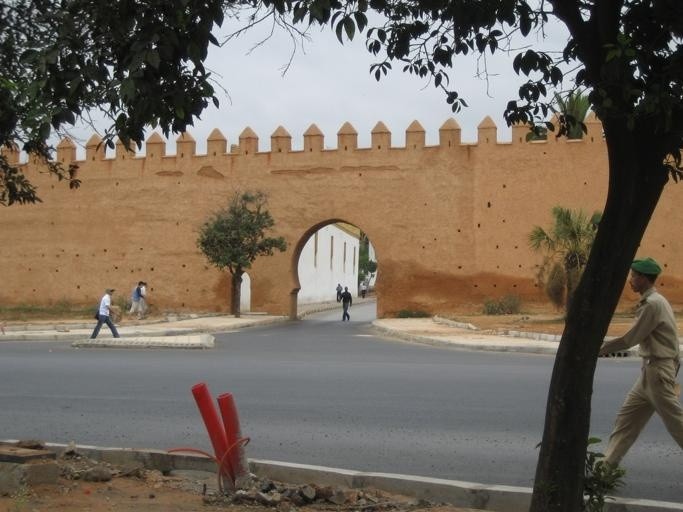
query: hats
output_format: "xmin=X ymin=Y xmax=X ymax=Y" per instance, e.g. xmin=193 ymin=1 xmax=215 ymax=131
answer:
xmin=631 ymin=257 xmax=661 ymax=274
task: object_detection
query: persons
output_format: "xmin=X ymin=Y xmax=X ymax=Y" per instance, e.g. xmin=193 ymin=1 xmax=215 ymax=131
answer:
xmin=359 ymin=282 xmax=366 ymax=299
xmin=134 ymin=281 xmax=147 ymax=320
xmin=335 ymin=283 xmax=342 ymax=303
xmin=127 ymin=281 xmax=147 ymax=320
xmin=582 ymin=257 xmax=683 ymax=496
xmin=88 ymin=288 xmax=120 ymax=339
xmin=339 ymin=286 xmax=352 ymax=322
